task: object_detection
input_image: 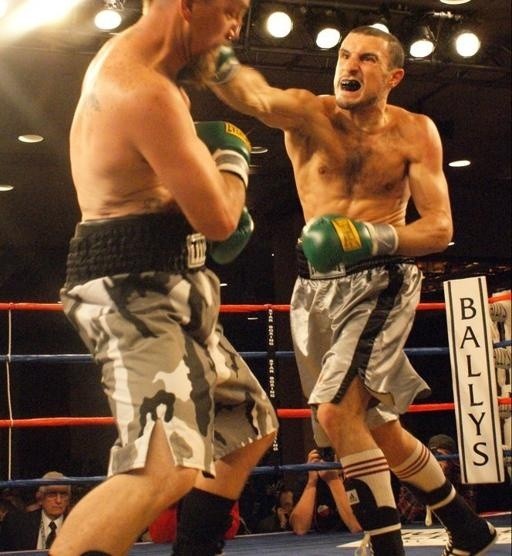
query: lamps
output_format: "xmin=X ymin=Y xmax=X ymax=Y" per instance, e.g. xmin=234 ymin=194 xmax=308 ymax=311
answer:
xmin=93 ymin=1 xmax=122 ymax=31
xmin=408 ymin=25 xmax=438 ymax=59
xmin=313 ymin=13 xmax=343 ymax=50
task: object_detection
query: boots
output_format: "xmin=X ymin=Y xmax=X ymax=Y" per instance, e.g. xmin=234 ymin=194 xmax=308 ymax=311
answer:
xmin=170 ymin=488 xmax=237 ymax=556
xmin=357 ymin=506 xmax=406 ymax=556
xmin=420 ymin=480 xmax=499 ymax=556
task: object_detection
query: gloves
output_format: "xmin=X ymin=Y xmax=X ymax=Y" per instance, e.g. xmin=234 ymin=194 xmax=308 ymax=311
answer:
xmin=301 ymin=214 xmax=399 ymax=273
xmin=206 ymin=207 xmax=255 ymax=264
xmin=177 ymin=43 xmax=239 ymax=87
xmin=194 ymin=120 xmax=252 ymax=189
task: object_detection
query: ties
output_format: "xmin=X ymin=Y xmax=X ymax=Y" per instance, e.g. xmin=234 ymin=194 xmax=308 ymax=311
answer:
xmin=45 ymin=521 xmax=57 ymax=549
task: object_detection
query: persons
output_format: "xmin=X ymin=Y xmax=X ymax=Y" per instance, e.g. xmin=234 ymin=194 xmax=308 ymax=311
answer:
xmin=180 ymin=25 xmax=499 ymax=556
xmin=49 ymin=0 xmax=280 ymax=555
xmin=1 ymin=432 xmax=477 ymax=555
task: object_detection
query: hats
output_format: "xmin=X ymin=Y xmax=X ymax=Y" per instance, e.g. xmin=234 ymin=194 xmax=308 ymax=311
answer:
xmin=428 ymin=434 xmax=458 ymax=453
